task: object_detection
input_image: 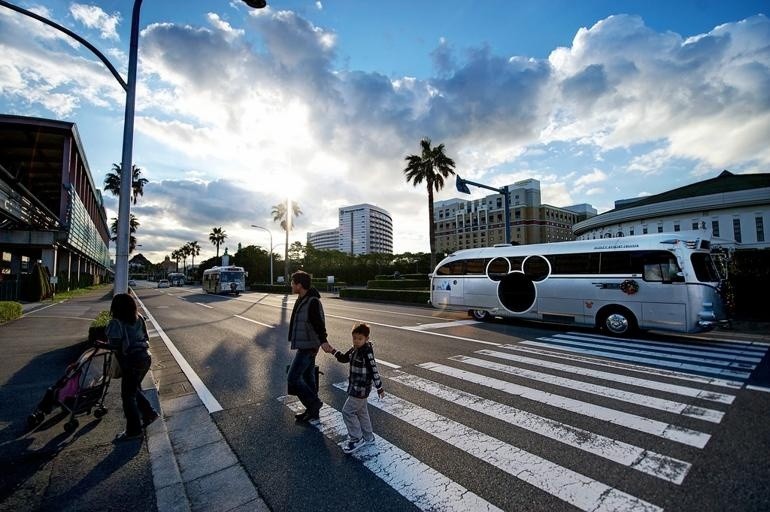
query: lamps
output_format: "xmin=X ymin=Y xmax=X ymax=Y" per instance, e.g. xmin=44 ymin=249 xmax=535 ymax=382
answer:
xmin=37 ymin=256 xmax=43 ymax=265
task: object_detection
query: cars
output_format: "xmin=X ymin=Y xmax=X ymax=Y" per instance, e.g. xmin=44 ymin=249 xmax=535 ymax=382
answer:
xmin=157 ymin=278 xmax=170 ymax=289
xmin=127 ymin=279 xmax=135 ymax=286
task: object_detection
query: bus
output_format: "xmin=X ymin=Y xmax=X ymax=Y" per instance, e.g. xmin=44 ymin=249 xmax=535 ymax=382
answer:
xmin=200 ymin=265 xmax=246 ymax=296
xmin=167 ymin=272 xmax=185 ymax=287
xmin=424 ymin=241 xmax=730 ymax=339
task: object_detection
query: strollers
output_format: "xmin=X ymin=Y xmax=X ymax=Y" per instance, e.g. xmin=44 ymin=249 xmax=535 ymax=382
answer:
xmin=32 ymin=337 xmax=114 ymax=435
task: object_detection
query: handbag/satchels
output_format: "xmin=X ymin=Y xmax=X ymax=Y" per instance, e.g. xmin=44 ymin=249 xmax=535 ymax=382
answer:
xmin=286 ymin=365 xmax=325 ymax=393
xmin=107 ymin=352 xmax=123 ymax=380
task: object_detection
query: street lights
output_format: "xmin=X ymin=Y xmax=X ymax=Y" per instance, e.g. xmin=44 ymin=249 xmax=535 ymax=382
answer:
xmin=134 ymin=244 xmax=142 ymax=248
xmin=456 ymin=174 xmax=511 ymax=244
xmin=0 ymin=0 xmax=269 ymax=326
xmin=251 ymin=225 xmax=274 ymax=284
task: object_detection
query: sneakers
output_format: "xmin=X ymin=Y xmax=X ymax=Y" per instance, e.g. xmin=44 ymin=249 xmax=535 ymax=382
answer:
xmin=114 ymin=430 xmax=145 ymax=442
xmin=362 ymin=436 xmax=376 ymax=445
xmin=342 ymin=437 xmax=366 ymax=454
xmin=142 ymin=407 xmax=160 ymax=427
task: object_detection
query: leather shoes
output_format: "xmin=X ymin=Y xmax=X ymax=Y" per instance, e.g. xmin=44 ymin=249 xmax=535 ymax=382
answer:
xmin=302 ymin=399 xmax=323 ymax=421
xmin=295 ymin=411 xmax=319 ymax=419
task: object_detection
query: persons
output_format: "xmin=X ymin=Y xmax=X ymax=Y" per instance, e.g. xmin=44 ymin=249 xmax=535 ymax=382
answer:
xmin=329 ymin=321 xmax=385 ymax=451
xmin=93 ymin=294 xmax=160 ymax=440
xmin=58 ymin=360 xmax=78 ymax=410
xmin=286 ymin=271 xmax=330 ymax=423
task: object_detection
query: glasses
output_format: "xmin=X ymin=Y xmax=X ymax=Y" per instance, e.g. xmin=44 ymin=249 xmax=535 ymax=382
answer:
xmin=290 ymin=281 xmax=297 ymax=286
